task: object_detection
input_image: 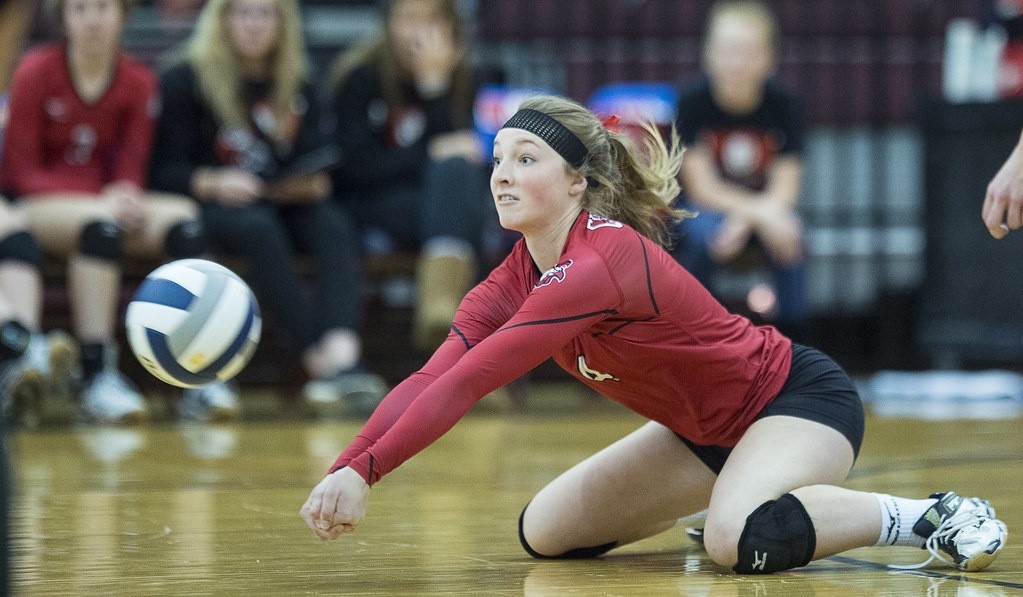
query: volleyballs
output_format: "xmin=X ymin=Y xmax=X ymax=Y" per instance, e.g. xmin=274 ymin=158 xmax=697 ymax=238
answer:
xmin=125 ymin=259 xmax=262 ymax=390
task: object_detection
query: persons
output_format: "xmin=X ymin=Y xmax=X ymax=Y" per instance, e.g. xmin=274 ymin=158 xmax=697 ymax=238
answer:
xmin=299 ymin=95 xmax=1009 ymax=573
xmin=0 ymin=0 xmax=1023 ymax=429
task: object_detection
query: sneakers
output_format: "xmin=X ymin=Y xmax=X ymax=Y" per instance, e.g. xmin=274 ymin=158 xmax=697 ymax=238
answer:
xmin=887 ymin=490 xmax=1008 ymax=573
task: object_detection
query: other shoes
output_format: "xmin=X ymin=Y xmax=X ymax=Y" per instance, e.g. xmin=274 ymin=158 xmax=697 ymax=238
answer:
xmin=303 ymin=366 xmax=385 ymax=415
xmin=175 ymin=383 xmax=243 ymax=423
xmin=82 ymin=371 xmax=147 ymax=424
xmin=3 ymin=331 xmax=83 ymax=428
